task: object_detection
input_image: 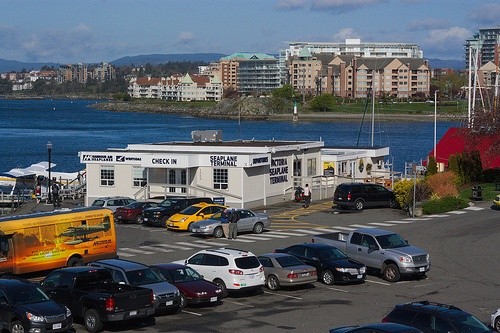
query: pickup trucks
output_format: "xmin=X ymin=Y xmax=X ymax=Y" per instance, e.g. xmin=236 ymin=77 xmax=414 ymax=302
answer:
xmin=312 ymin=228 xmax=431 ymax=282
xmin=37 ymin=266 xmax=154 ymax=333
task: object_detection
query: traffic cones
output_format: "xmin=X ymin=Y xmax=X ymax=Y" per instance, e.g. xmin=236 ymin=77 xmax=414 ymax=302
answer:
xmin=434 ymin=90 xmax=440 ymax=159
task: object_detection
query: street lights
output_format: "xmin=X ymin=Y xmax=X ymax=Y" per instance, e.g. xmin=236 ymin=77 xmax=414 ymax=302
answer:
xmin=46 ymin=141 xmax=53 ymax=204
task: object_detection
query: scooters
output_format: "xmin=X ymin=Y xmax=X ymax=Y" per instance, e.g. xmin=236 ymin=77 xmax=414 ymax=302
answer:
xmin=294 ymin=186 xmax=305 ymax=202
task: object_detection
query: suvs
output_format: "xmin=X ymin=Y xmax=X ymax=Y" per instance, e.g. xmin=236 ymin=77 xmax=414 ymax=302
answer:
xmin=382 ymin=300 xmax=500 ymax=333
xmin=143 ymin=196 xmax=214 ymax=228
xmin=0 ymin=274 xmax=73 ymax=333
xmin=170 ymin=247 xmax=265 ymax=298
xmin=86 ymin=258 xmax=181 ymax=317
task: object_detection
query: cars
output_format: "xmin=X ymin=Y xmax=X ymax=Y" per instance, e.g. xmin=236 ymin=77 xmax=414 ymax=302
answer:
xmin=189 ymin=208 xmax=271 ymax=238
xmin=490 ymin=308 xmax=500 ymax=332
xmin=330 ymin=322 xmax=424 ymax=333
xmin=151 ymin=263 xmax=223 ymax=310
xmin=333 ymin=183 xmax=398 ymax=210
xmin=92 ymin=197 xmax=145 ymax=224
xmin=493 ymin=194 xmax=500 ymax=206
xmin=274 ymin=243 xmax=367 ymax=285
xmin=258 ymin=254 xmax=317 ymax=291
xmin=166 ymin=202 xmax=229 ymax=232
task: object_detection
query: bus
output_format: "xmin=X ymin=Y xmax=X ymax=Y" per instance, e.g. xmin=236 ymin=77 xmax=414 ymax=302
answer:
xmin=0 ymin=207 xmax=117 ymax=274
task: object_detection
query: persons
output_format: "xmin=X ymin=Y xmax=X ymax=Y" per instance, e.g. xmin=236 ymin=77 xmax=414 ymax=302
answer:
xmin=52 ymin=183 xmax=60 ymax=207
xmin=221 ymin=208 xmax=230 ymax=239
xmin=227 ymin=208 xmax=240 ymax=239
xmin=302 ymin=184 xmax=312 ymax=208
